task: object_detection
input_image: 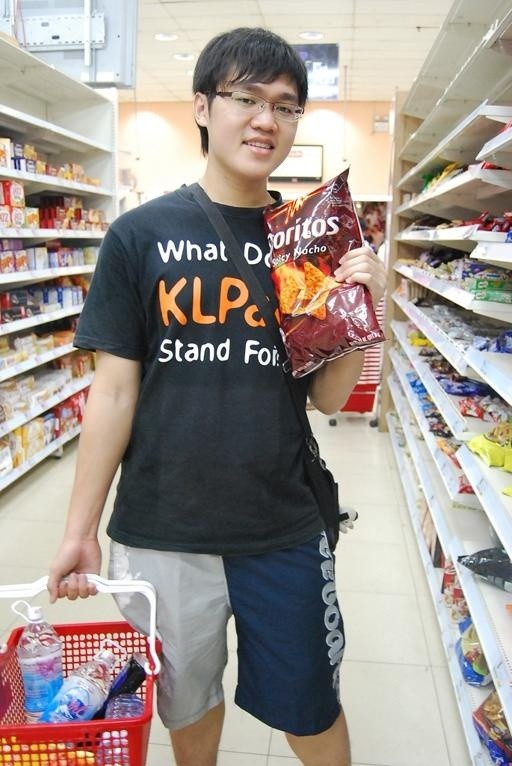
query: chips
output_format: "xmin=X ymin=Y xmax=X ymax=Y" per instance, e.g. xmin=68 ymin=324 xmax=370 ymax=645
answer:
xmin=275 ymin=262 xmax=341 ymax=320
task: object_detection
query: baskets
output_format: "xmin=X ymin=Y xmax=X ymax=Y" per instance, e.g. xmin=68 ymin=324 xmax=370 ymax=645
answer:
xmin=0 ymin=565 xmax=165 ymax=766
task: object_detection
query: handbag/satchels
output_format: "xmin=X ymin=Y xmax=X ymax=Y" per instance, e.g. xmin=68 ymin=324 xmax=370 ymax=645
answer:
xmin=297 ymin=431 xmax=341 ymax=552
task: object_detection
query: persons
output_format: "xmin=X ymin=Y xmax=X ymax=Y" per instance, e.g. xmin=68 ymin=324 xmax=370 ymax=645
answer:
xmin=47 ymin=22 xmax=387 ymax=766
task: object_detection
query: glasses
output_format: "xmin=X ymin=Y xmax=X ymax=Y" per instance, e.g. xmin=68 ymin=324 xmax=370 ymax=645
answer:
xmin=215 ymin=83 xmax=306 ymax=123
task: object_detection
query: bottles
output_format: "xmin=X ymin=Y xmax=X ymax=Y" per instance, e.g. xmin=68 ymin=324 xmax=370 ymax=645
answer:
xmin=12 ymin=599 xmax=63 ymax=723
xmin=100 ymin=692 xmax=144 ymax=765
xmin=39 ymin=648 xmax=118 ymax=728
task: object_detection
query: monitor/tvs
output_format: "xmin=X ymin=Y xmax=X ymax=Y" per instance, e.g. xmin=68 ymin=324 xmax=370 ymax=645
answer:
xmin=1 ymin=0 xmax=139 ymax=89
xmin=268 ymin=145 xmax=323 ymax=182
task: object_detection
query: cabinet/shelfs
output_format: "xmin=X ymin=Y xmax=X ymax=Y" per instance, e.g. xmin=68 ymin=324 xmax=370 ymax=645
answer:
xmin=0 ymin=34 xmax=120 ymax=492
xmin=376 ymin=1 xmax=512 ymax=766
